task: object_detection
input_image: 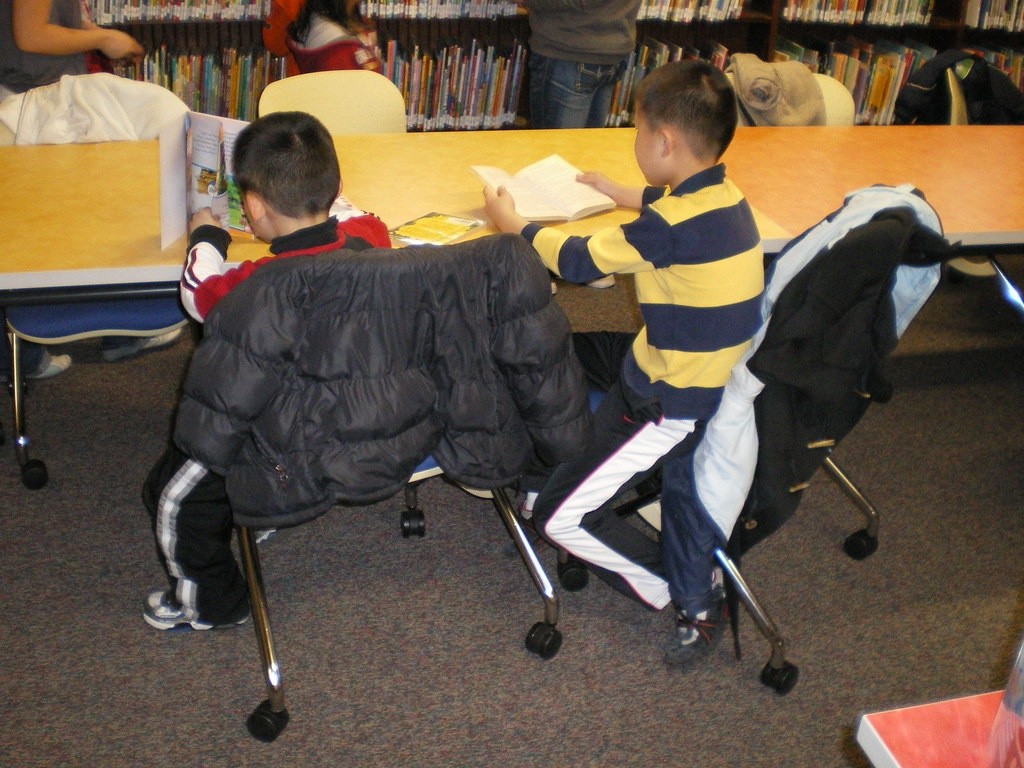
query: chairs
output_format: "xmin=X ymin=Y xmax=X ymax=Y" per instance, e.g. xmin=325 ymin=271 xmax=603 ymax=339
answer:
xmin=0 ymin=71 xmax=191 ymax=491
xmin=256 ymin=68 xmax=407 ymax=135
xmin=173 ymin=230 xmax=596 ymax=743
xmin=722 ymin=51 xmax=856 ymax=127
xmin=556 ymin=183 xmax=963 ymax=696
xmin=892 ymin=48 xmax=1024 ymax=276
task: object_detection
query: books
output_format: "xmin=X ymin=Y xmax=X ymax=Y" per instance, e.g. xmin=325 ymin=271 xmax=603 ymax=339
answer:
xmin=771 ymin=32 xmax=937 ymax=126
xmin=376 ymin=25 xmax=526 ymax=131
xmin=108 ymin=43 xmax=287 ymax=122
xmin=783 ymin=0 xmax=936 ymax=26
xmin=389 ymin=212 xmax=487 ymax=247
xmin=959 ymin=0 xmax=1024 ymax=34
xmin=468 ymin=152 xmax=617 ymax=222
xmin=636 ymin=0 xmax=744 ymax=22
xmin=960 ymin=44 xmax=1024 ymax=95
xmin=605 ymin=37 xmax=730 ymax=129
xmin=88 ymin=0 xmax=271 ymax=26
xmin=158 ymin=111 xmax=254 ymax=252
xmin=358 ymin=0 xmax=518 ymax=20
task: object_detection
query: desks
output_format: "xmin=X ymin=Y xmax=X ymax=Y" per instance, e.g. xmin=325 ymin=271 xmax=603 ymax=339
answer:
xmin=1 ymin=124 xmax=1024 ymax=318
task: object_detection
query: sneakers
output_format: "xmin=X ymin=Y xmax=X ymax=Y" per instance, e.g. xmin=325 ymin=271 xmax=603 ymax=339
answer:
xmin=664 ymin=572 xmax=729 ymax=667
xmin=141 ymin=588 xmax=250 ymax=633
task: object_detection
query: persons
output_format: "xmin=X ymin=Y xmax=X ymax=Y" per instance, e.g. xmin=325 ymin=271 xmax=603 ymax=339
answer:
xmin=0 ymin=83 xmax=182 ymax=380
xmin=523 ymin=0 xmax=641 ymax=130
xmin=0 ymin=0 xmax=146 ymax=96
xmin=466 ymin=58 xmax=766 ymax=669
xmin=286 ymin=0 xmax=383 ymax=75
xmin=140 ymin=108 xmax=391 ymax=633
xmin=263 ymin=0 xmax=362 ymax=78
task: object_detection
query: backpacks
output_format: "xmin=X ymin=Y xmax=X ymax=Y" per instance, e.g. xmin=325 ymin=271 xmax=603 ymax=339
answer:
xmin=895 ymin=47 xmax=1022 ymax=124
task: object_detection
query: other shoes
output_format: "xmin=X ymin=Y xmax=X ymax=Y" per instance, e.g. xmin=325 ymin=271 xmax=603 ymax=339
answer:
xmin=26 ymin=351 xmax=72 ymax=379
xmin=103 ymin=329 xmax=184 ymax=360
